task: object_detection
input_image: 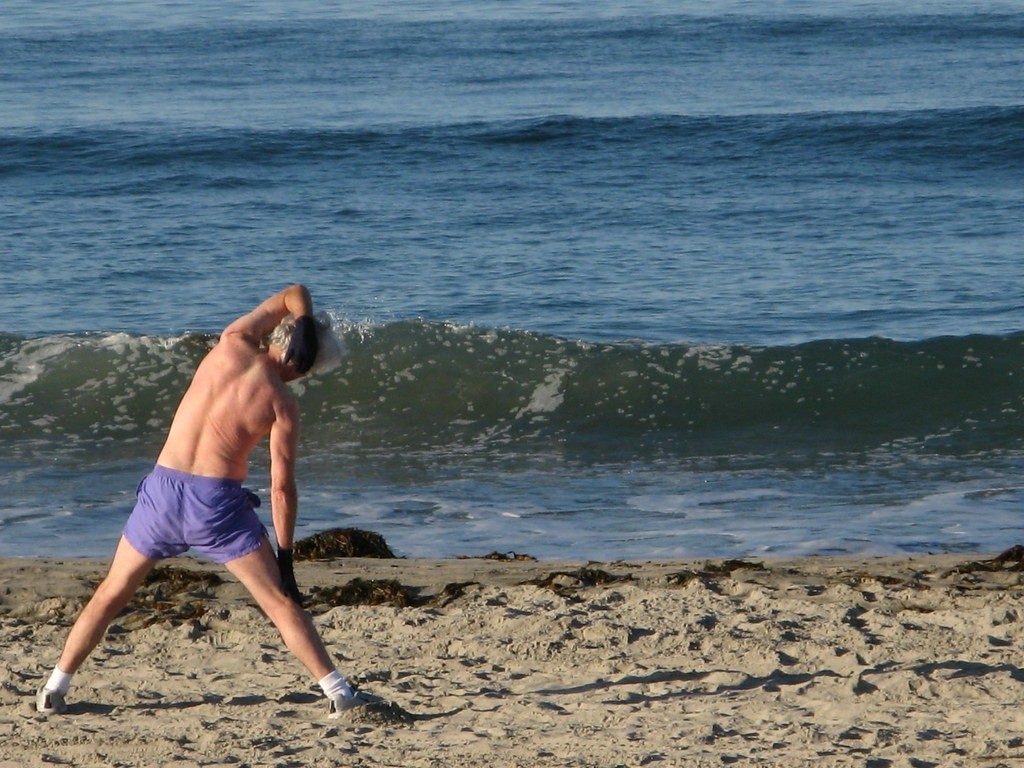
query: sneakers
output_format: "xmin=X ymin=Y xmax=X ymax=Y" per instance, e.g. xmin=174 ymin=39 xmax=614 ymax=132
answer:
xmin=328 ymin=679 xmax=385 ymax=719
xmin=37 ymin=683 xmax=67 ymax=714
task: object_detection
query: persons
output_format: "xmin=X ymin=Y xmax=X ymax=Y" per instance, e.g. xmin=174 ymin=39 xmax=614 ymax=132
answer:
xmin=35 ymin=284 xmax=385 ymax=719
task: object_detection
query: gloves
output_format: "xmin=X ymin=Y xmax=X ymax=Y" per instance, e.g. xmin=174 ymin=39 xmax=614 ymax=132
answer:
xmin=282 ymin=316 xmax=319 ymax=374
xmin=276 ymin=544 xmax=304 ymax=609
